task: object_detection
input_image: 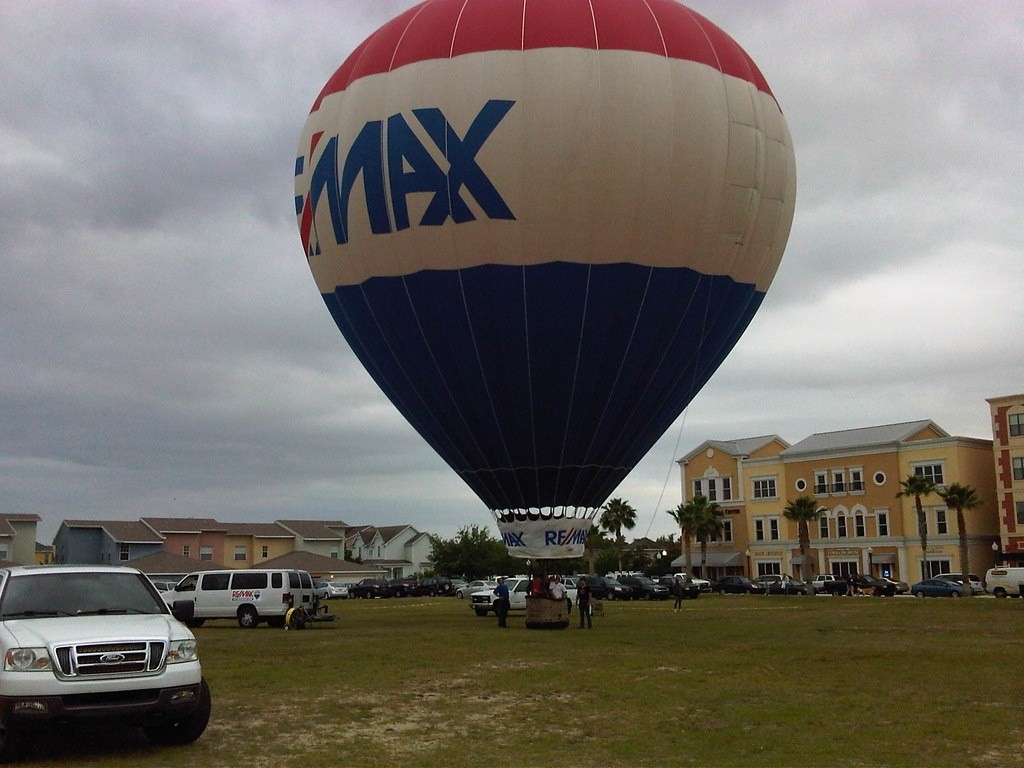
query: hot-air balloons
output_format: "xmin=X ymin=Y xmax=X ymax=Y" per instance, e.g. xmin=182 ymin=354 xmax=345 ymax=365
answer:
xmin=288 ymin=0 xmax=799 ymax=633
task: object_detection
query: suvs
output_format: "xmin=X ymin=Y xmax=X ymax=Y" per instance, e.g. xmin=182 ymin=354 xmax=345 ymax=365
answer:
xmin=0 ymin=564 xmax=213 ymax=755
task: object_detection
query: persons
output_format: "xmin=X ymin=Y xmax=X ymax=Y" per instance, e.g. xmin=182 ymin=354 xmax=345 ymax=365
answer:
xmin=493 ymin=577 xmax=511 ymax=629
xmin=672 ymin=578 xmax=685 ymax=613
xmin=525 ymin=574 xmax=567 ymax=601
xmin=846 ymin=571 xmax=861 ymax=597
xmin=781 ymin=572 xmax=790 ymax=596
xmin=575 ymin=577 xmax=593 ymax=629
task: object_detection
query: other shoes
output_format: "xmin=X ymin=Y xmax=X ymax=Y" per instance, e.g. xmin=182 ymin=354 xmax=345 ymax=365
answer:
xmin=679 ymin=609 xmax=682 ymax=612
xmin=588 ymin=622 xmax=592 ymax=630
xmin=578 ymin=624 xmax=584 ymax=630
xmin=674 ymin=609 xmax=677 ymax=612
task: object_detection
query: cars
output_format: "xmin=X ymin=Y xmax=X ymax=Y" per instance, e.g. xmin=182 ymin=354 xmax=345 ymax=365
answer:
xmin=309 ymin=556 xmax=1024 ymax=604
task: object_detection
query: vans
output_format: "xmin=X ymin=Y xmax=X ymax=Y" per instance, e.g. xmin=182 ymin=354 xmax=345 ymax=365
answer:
xmin=156 ymin=568 xmax=317 ymax=629
